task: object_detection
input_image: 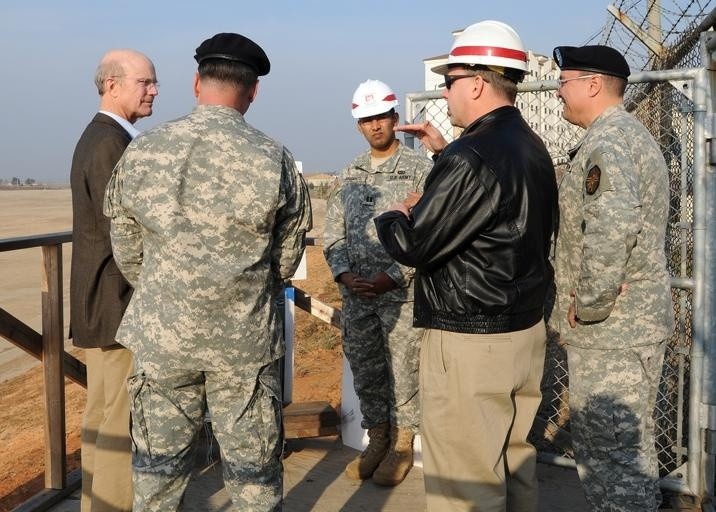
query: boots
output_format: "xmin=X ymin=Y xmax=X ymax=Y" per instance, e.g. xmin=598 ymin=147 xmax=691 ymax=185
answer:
xmin=345 ymin=424 xmax=390 ymax=479
xmin=372 ymin=427 xmax=415 ymax=487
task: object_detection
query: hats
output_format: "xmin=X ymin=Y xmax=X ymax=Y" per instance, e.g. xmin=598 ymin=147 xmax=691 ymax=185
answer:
xmin=194 ymin=32 xmax=270 ymax=77
xmin=553 ymin=45 xmax=631 ymax=80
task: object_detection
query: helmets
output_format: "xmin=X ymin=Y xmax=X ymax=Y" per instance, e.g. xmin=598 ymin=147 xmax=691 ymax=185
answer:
xmin=352 ymin=79 xmax=400 ymax=119
xmin=430 ymin=20 xmax=531 ymax=75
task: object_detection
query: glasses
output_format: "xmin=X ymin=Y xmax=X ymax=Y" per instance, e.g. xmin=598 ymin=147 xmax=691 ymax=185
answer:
xmin=558 ymin=72 xmax=601 ymax=87
xmin=446 ymin=75 xmax=490 ymax=89
xmin=109 ymin=75 xmax=161 ymax=89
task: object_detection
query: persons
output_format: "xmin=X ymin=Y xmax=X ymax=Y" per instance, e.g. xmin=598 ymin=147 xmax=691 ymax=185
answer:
xmin=323 ymin=79 xmax=433 ymax=488
xmin=372 ymin=17 xmax=563 ymax=512
xmin=547 ymin=41 xmax=678 ymax=512
xmin=67 ymin=48 xmax=160 ymax=512
xmin=103 ymin=33 xmax=313 ymax=512
